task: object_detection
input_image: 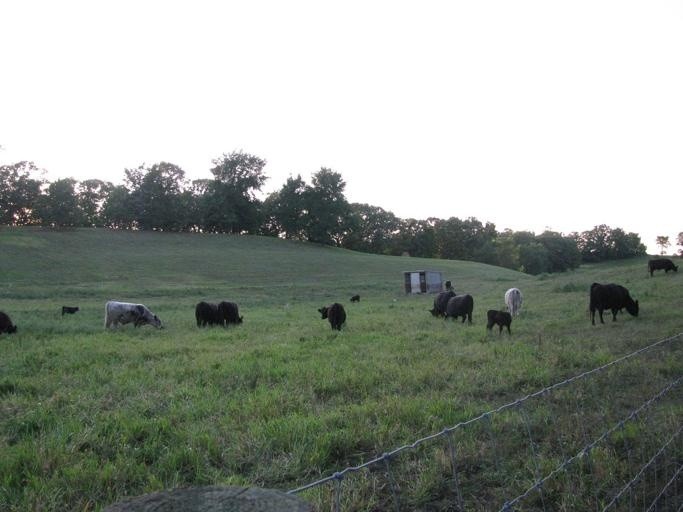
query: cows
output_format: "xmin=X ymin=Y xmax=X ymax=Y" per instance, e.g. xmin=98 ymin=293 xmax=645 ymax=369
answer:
xmin=318 ymin=302 xmax=346 ymax=331
xmin=486 ymin=309 xmax=512 ymax=337
xmin=444 ymin=291 xmax=474 ymax=323
xmin=505 ymin=287 xmax=523 ymax=317
xmin=195 ymin=300 xmax=218 ymax=329
xmin=648 ymin=258 xmax=679 ymax=277
xmin=445 ymin=280 xmax=453 ymax=290
xmin=104 ymin=300 xmax=163 ymax=329
xmin=589 ymin=282 xmax=640 ymax=327
xmin=217 ymin=301 xmax=243 ymax=328
xmin=350 ymin=294 xmax=360 ymax=303
xmin=428 ymin=291 xmax=456 ymax=319
xmin=62 ymin=305 xmax=80 ymax=316
xmin=0 ymin=312 xmax=17 ymax=335
